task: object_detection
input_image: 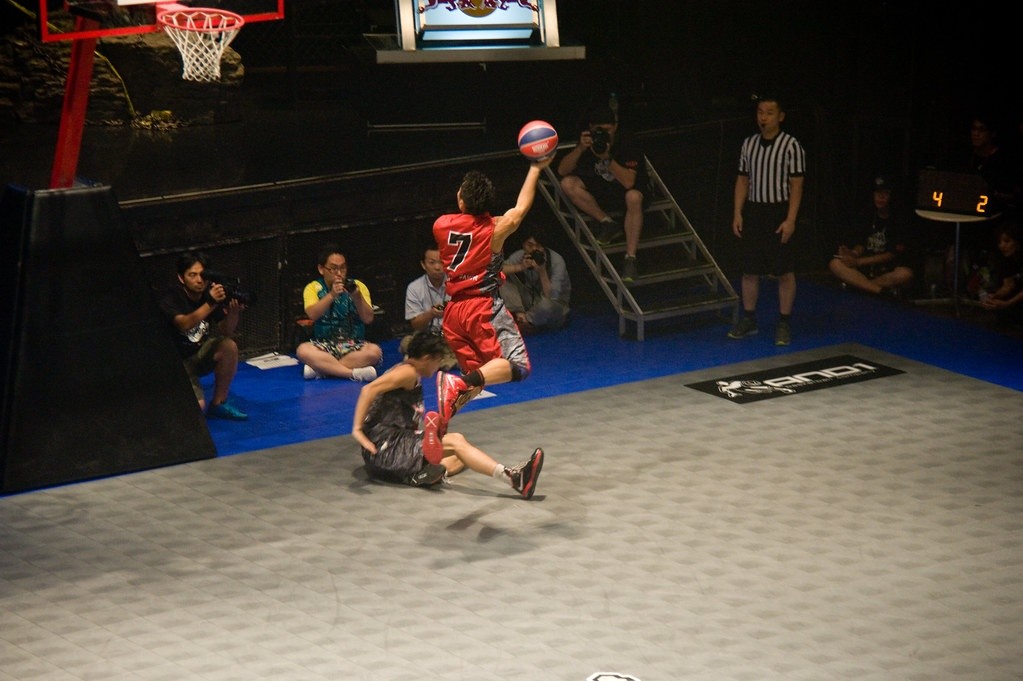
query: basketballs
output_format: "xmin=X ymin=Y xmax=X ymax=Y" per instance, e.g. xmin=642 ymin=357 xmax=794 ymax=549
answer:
xmin=518 ymin=120 xmax=558 ymax=162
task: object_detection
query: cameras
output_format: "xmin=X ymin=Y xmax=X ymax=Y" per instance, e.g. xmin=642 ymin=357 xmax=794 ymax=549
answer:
xmin=588 ymin=126 xmax=611 ymax=154
xmin=342 ymin=276 xmax=357 ymax=293
xmin=528 ymin=250 xmax=545 ymax=265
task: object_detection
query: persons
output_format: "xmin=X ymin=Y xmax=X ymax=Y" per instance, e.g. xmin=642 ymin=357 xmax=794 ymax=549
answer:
xmin=398 ymin=248 xmax=465 ymax=370
xmin=498 ymin=235 xmax=570 ymax=331
xmin=296 ymin=250 xmax=383 ymax=382
xmin=352 ymin=332 xmax=544 ymax=500
xmin=726 ymin=100 xmax=808 ymax=347
xmin=159 ymin=250 xmax=248 ymax=420
xmin=829 ymin=177 xmax=916 ymax=298
xmin=922 ymin=119 xmax=1023 ymax=319
xmin=421 ymin=154 xmax=558 ymax=465
xmin=557 ymin=111 xmax=652 ymax=285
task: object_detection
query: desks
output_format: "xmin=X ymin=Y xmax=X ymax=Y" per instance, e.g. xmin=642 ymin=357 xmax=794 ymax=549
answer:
xmin=911 ymin=209 xmax=1002 ymax=319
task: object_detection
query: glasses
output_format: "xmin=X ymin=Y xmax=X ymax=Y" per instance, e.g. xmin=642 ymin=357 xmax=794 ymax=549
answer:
xmin=323 ymin=266 xmax=348 ymax=273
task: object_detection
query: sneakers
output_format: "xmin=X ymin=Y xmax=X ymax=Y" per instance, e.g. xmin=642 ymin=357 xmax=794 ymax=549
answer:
xmin=352 ymin=366 xmax=377 ymax=381
xmin=410 ymin=464 xmax=447 ymax=487
xmin=728 ymin=317 xmax=759 ymax=339
xmin=622 ymin=256 xmax=638 ymax=283
xmin=423 ymin=411 xmax=448 ymax=465
xmin=504 ymin=448 xmax=544 ymax=499
xmin=775 ymin=321 xmax=791 ymax=346
xmin=596 ymin=221 xmax=623 ymax=245
xmin=304 ymin=364 xmax=321 ymax=379
xmin=437 ymin=370 xmax=468 ymax=418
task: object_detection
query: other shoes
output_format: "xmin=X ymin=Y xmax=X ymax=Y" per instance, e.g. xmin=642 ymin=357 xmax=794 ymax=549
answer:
xmin=839 ymin=282 xmax=863 ymax=295
xmin=518 ymin=321 xmax=537 ymax=335
xmin=887 ymin=285 xmax=903 ymax=305
xmin=205 ymin=401 xmax=247 ymax=420
